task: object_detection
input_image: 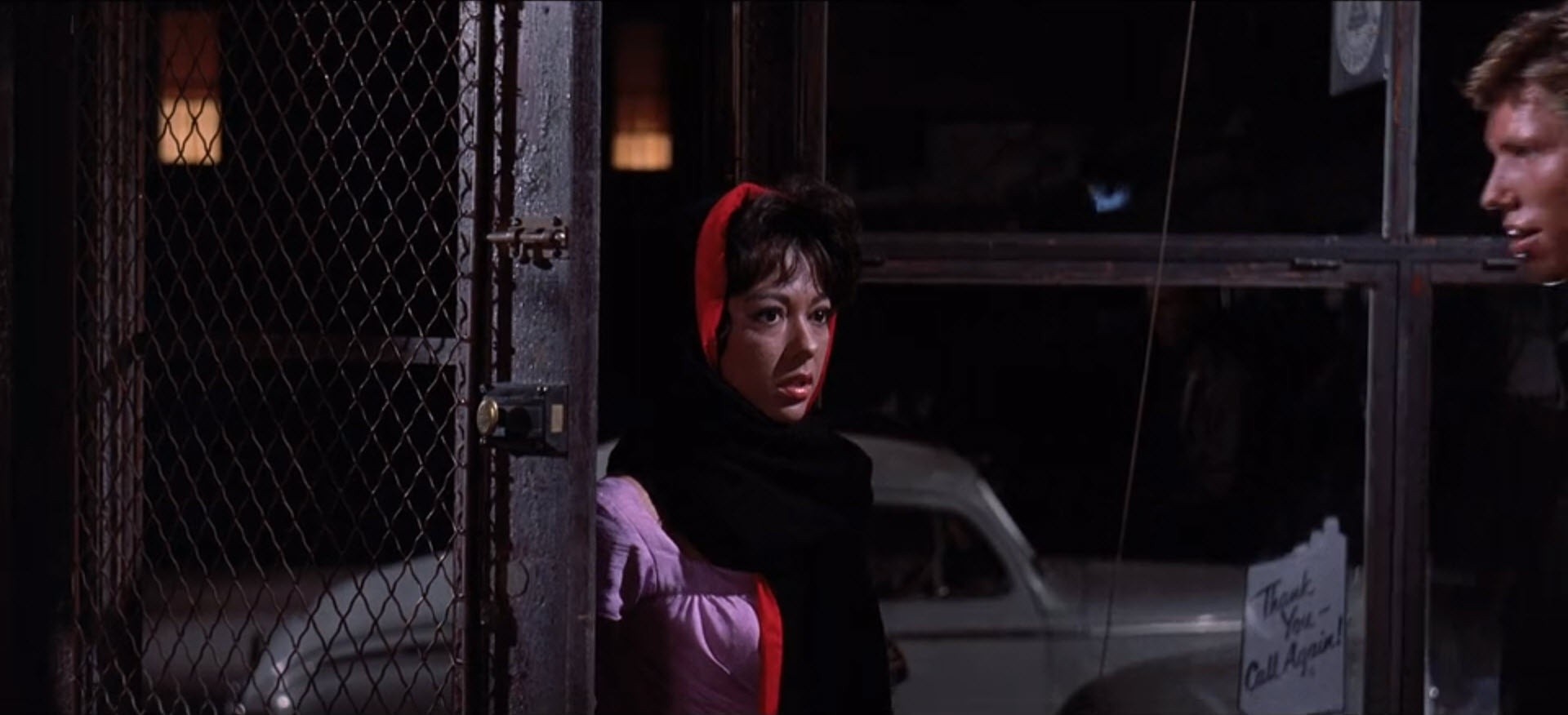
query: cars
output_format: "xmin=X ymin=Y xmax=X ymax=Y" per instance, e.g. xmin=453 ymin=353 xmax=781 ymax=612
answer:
xmin=236 ymin=436 xmax=1365 ymax=715
xmin=138 ymin=574 xmax=336 ymax=690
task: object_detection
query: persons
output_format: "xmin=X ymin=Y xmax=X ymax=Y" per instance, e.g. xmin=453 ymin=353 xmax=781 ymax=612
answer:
xmin=1462 ymin=4 xmax=1568 ymax=715
xmin=596 ymin=175 xmax=911 ymax=715
